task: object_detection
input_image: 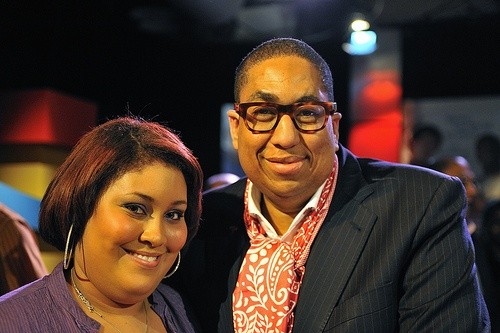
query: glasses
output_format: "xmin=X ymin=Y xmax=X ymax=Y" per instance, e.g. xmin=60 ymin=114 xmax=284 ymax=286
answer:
xmin=233 ymin=100 xmax=338 ymax=134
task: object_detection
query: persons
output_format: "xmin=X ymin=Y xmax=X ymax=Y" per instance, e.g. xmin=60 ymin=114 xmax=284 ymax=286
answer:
xmin=0 ymin=117 xmax=203 ymax=333
xmin=171 ymin=38 xmax=500 ymax=333
xmin=400 ymin=121 xmax=500 ymax=333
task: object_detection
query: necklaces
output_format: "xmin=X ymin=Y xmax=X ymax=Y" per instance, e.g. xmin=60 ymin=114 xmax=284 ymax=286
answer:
xmin=71 ymin=268 xmax=148 ymax=333
